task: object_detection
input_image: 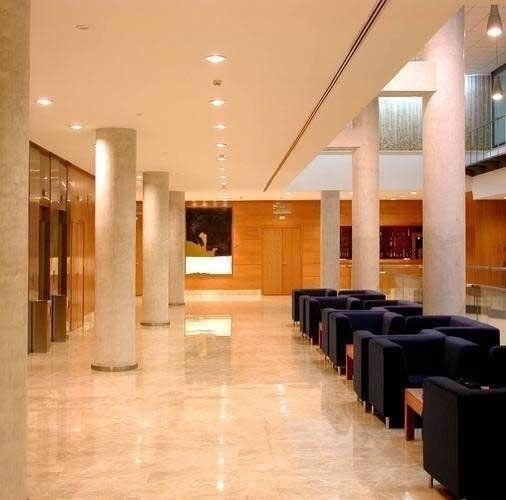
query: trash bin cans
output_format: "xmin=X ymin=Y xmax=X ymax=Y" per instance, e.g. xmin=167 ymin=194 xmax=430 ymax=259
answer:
xmin=33 ymin=300 xmax=51 ymax=352
xmin=53 ymin=295 xmax=68 ymax=342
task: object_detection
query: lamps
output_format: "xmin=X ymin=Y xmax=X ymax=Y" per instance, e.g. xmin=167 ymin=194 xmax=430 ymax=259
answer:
xmin=492 ymin=38 xmax=503 ymax=101
xmin=486 ymin=0 xmax=503 ymax=38
